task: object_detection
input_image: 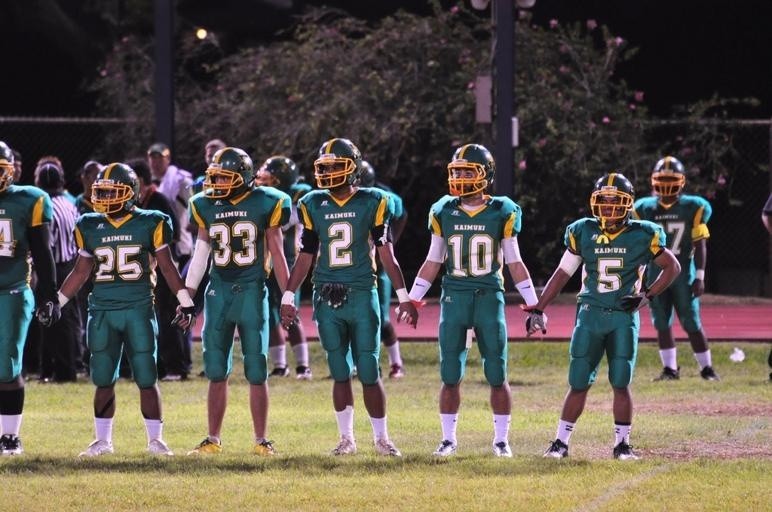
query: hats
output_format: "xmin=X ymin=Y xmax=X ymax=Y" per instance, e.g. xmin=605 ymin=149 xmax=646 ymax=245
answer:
xmin=146 ymin=141 xmax=173 ymax=158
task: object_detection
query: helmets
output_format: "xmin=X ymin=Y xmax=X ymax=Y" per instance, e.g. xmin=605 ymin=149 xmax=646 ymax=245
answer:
xmin=258 ymin=155 xmax=299 ymax=192
xmin=0 ymin=138 xmax=16 ymax=193
xmin=651 ymin=153 xmax=687 ymax=197
xmin=206 ymin=146 xmax=257 ymax=200
xmin=588 ymin=172 xmax=636 ymax=227
xmin=88 ymin=161 xmax=142 ymax=216
xmin=314 ymin=136 xmax=363 ymax=192
xmin=356 ymin=159 xmax=376 ymax=189
xmin=446 ymin=142 xmax=497 ymax=196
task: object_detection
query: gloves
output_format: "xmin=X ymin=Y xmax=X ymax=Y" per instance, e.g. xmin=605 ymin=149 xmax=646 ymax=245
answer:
xmin=621 ymin=289 xmax=655 ymax=315
xmin=168 ymin=305 xmax=198 ymax=339
xmin=36 ymin=297 xmax=61 ymax=324
xmin=393 ymin=298 xmax=418 ymax=327
xmin=44 ymin=296 xmax=63 ymax=329
xmin=524 ymin=311 xmax=546 ymax=338
xmin=525 ymin=313 xmax=549 ymax=330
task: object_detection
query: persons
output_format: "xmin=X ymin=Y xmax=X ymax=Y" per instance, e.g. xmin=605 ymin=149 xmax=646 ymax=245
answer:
xmin=75 ymin=161 xmax=105 ymax=378
xmin=761 ymin=191 xmax=772 ymax=384
xmin=630 ymin=156 xmax=720 ymax=382
xmin=12 ymin=149 xmax=23 ymax=183
xmin=255 ymin=156 xmax=313 ymax=379
xmin=280 ymin=138 xmax=408 ymax=458
xmin=525 ymin=173 xmax=683 ymax=461
xmin=192 ymin=139 xmax=229 ymax=195
xmin=126 ymin=159 xmax=193 ymax=381
xmin=393 ymin=144 xmax=548 ymax=459
xmin=170 ymin=145 xmax=300 ymax=459
xmin=0 ymin=138 xmax=62 ymax=456
xmin=147 ymin=143 xmax=194 ymax=282
xmin=35 ymin=162 xmax=195 ymax=460
xmin=352 ymin=160 xmax=414 ymax=380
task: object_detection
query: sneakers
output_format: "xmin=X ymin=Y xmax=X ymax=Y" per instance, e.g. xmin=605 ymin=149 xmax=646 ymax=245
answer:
xmin=147 ymin=438 xmax=175 ymax=457
xmin=542 ymin=439 xmax=570 ymax=461
xmin=431 ymin=439 xmax=458 ymax=457
xmin=295 ymin=366 xmax=313 ymax=380
xmin=161 ymin=368 xmax=184 ymax=381
xmin=699 ymin=365 xmax=721 ymax=383
xmin=373 ymin=439 xmax=402 ymax=459
xmin=492 ymin=440 xmax=515 ymax=460
xmin=388 ymin=362 xmax=406 ymax=380
xmin=612 ymin=442 xmax=640 ymax=461
xmin=253 ymin=439 xmax=277 ymax=457
xmin=78 ymin=441 xmax=115 ymax=459
xmin=185 ymin=436 xmax=223 ymax=456
xmin=1 ymin=432 xmax=23 ymax=455
xmin=267 ymin=364 xmax=292 ymax=381
xmin=650 ymin=365 xmax=681 ymax=383
xmin=331 ymin=438 xmax=359 ymax=457
